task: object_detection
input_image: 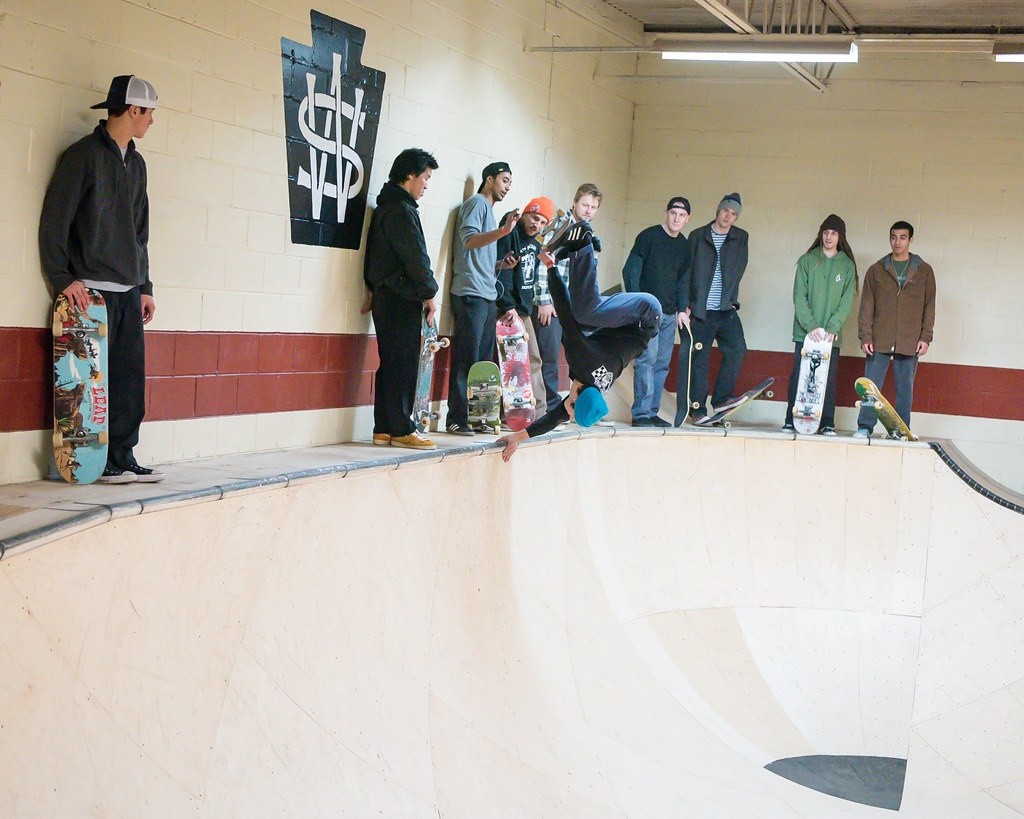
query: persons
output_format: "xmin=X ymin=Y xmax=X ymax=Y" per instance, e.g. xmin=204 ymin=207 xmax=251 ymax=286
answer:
xmin=445 ymin=161 xmax=521 ymax=435
xmin=622 ymin=197 xmax=690 ymax=427
xmin=495 ymin=195 xmax=565 ymax=431
xmin=39 ymin=75 xmax=164 ymax=484
xmin=529 ymin=183 xmax=616 ymax=427
xmin=495 ymin=219 xmax=661 ymax=463
xmin=360 ymin=147 xmax=439 ymax=450
xmin=850 ymin=221 xmax=936 ymax=440
xmin=688 ymin=192 xmax=748 ymax=424
xmin=782 ymin=214 xmax=859 ymax=436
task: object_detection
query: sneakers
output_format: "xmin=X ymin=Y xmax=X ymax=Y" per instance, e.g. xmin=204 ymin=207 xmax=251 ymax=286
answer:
xmin=820 ymin=427 xmax=837 ymax=435
xmin=390 ymin=429 xmax=437 ymax=449
xmin=650 ymin=416 xmax=671 ymax=427
xmin=122 ymin=463 xmax=165 ymax=482
xmin=373 ymin=433 xmax=391 ymax=445
xmin=691 ymin=414 xmax=710 ymax=424
xmin=852 ymin=429 xmax=870 ymax=438
xmin=782 ymin=424 xmax=797 ymax=433
xmin=96 ymin=464 xmax=137 ymax=483
xmin=547 ymin=218 xmax=593 ymax=255
xmin=554 ymin=237 xmax=601 ymax=260
xmin=446 ymin=422 xmax=475 ymax=436
xmin=713 ymin=395 xmax=748 ymax=413
xmin=632 ymin=418 xmax=653 ymax=427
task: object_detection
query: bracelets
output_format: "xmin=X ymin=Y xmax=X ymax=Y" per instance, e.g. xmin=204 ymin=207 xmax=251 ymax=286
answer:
xmin=502 ymin=226 xmax=508 ymax=235
xmin=546 ymin=260 xmax=554 ymax=266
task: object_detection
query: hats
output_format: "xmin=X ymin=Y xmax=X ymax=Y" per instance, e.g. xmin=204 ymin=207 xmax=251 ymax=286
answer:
xmin=477 ymin=162 xmax=512 ymax=193
xmin=91 ymin=75 xmax=157 ymax=109
xmin=820 ymin=214 xmax=845 ymax=236
xmin=666 ymin=197 xmax=690 ymax=215
xmin=522 ymin=195 xmax=554 ymax=225
xmin=716 ymin=192 xmax=742 ymax=221
xmin=574 ymin=387 xmax=608 ymax=427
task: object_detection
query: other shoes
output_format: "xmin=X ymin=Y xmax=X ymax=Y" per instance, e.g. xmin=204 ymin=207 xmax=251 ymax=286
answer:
xmin=597 ymin=419 xmax=615 ymax=426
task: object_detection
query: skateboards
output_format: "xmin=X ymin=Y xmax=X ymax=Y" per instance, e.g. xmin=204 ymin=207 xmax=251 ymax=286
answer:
xmin=495 ymin=314 xmax=535 ymax=431
xmin=413 ymin=307 xmax=451 ymax=434
xmin=466 ymin=359 xmax=504 ymax=436
xmin=792 ymin=327 xmax=838 ymax=434
xmin=674 ymin=320 xmax=703 ymax=428
xmin=534 ymin=208 xmax=572 ymax=247
xmin=699 ymin=377 xmax=776 ymax=429
xmin=854 ymin=377 xmax=916 ymax=442
xmin=52 ymin=288 xmax=110 ymax=486
